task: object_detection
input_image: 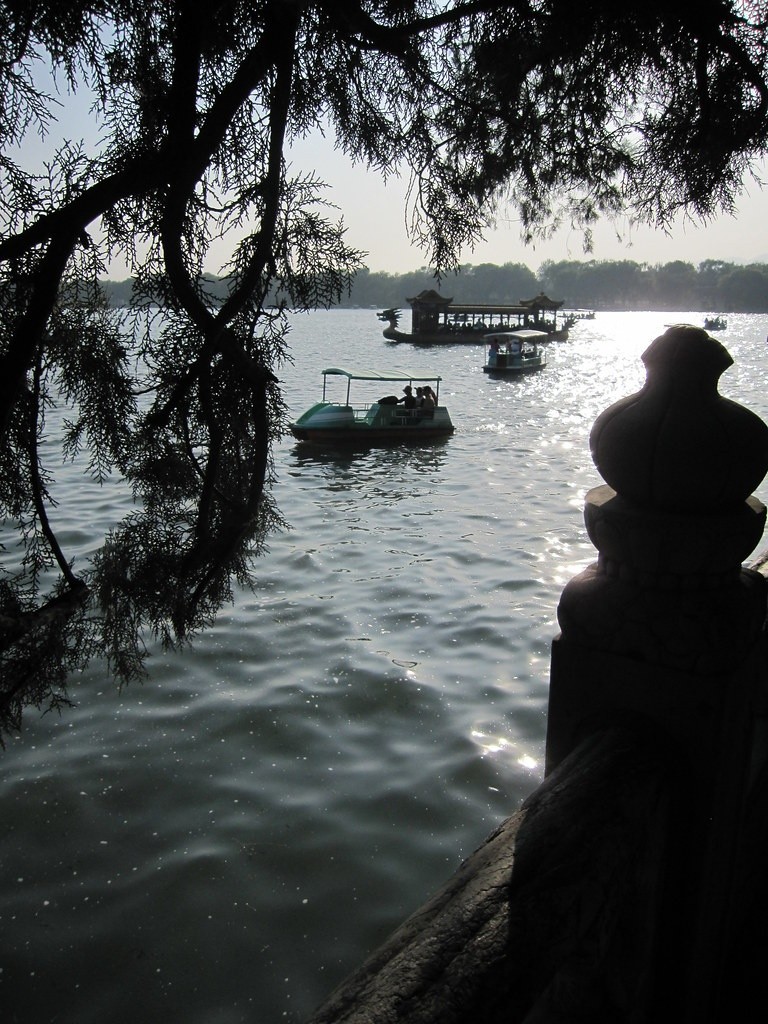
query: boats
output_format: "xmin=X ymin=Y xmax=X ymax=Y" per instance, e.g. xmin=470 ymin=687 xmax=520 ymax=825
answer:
xmin=480 ymin=329 xmax=550 ymax=376
xmin=706 ymin=312 xmax=729 ymax=330
xmin=376 ymin=289 xmax=578 ymax=345
xmin=282 ymin=366 xmax=460 ymax=444
xmin=559 ymin=305 xmax=597 ymax=319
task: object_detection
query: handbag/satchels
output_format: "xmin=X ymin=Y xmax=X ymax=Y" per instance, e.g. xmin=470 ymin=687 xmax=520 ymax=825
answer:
xmin=377 ymin=396 xmax=398 ymax=405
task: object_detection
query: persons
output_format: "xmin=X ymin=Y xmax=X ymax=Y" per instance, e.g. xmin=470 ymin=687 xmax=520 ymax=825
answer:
xmin=398 ymin=386 xmax=434 ymax=408
xmin=715 ymin=317 xmax=719 ymax=323
xmin=491 ymin=339 xmax=500 ymax=352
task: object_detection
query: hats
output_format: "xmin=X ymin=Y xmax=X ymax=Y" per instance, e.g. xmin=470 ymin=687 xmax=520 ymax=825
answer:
xmin=403 ymin=386 xmax=412 ymax=394
xmin=414 ymin=387 xmax=424 ymax=392
xmin=509 ymin=339 xmax=519 ymax=345
xmin=424 ymin=386 xmax=431 ymax=393
